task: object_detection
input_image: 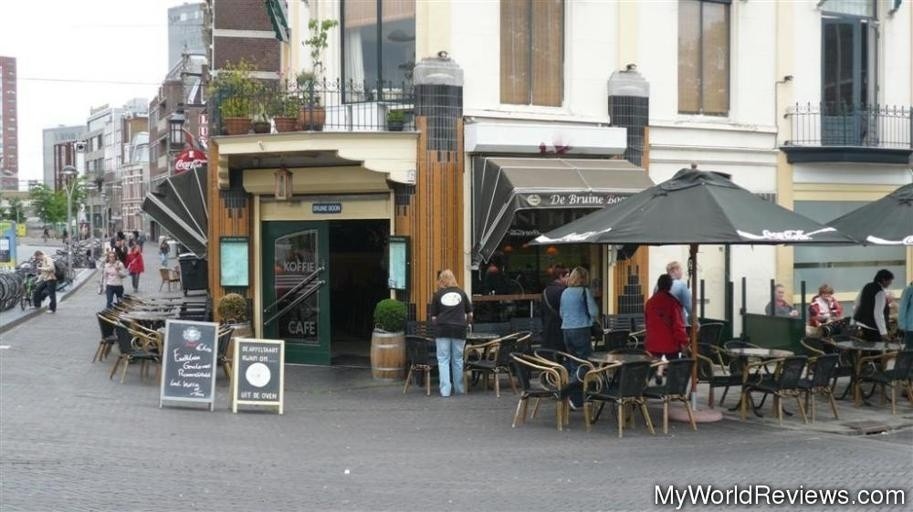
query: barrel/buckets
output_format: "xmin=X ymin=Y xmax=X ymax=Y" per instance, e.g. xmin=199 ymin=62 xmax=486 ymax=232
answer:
xmin=224 ymin=321 xmax=255 ymax=380
xmin=370 ymin=328 xmax=406 ymax=383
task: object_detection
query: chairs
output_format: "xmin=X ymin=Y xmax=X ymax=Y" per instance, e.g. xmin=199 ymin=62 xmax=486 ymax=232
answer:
xmin=92 ymin=292 xmax=240 ymax=390
xmin=401 ymin=308 xmax=912 ymax=444
xmin=158 ymin=267 xmax=181 ymax=293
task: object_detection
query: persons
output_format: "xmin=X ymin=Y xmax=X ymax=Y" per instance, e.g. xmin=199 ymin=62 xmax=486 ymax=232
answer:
xmin=652 ymin=261 xmax=701 ymax=348
xmin=541 ymin=264 xmax=571 ymax=382
xmin=559 ymin=267 xmax=600 ymax=410
xmin=763 ymin=284 xmax=799 ymax=317
xmin=852 ymin=270 xmax=894 ymax=342
xmin=22 ymin=225 xmax=175 ymax=314
xmin=809 ymin=284 xmax=842 ymax=330
xmin=430 ymin=268 xmax=473 ymax=398
xmin=896 ymin=282 xmax=912 ymax=398
xmin=644 ymin=274 xmax=690 ymax=359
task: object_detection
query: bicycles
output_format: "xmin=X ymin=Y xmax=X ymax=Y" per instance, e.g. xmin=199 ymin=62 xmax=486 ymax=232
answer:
xmin=0 ymin=238 xmax=102 ymax=310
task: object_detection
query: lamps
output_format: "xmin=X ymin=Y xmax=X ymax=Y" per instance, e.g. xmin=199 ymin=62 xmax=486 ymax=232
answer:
xmin=270 ymin=161 xmax=300 ymax=207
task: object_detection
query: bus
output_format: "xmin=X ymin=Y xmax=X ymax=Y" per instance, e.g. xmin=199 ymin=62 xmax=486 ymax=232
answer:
xmin=0 ymin=219 xmax=19 ymax=270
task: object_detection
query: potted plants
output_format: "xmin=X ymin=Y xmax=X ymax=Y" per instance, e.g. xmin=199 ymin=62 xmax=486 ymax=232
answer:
xmin=365 ymin=294 xmax=411 ymax=382
xmin=206 ymin=14 xmax=410 ymax=136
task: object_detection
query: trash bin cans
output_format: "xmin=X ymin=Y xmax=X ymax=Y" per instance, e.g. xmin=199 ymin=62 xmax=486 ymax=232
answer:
xmin=177 ymin=256 xmax=208 ymax=297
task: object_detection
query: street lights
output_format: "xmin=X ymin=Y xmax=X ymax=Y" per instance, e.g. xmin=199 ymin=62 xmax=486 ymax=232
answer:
xmin=72 ymin=141 xmax=88 ymax=238
xmin=84 ymin=182 xmax=98 ymax=269
xmin=104 ymin=196 xmax=110 ymax=242
xmin=100 ymin=193 xmax=106 ymax=242
xmin=59 ymin=163 xmax=78 ymax=279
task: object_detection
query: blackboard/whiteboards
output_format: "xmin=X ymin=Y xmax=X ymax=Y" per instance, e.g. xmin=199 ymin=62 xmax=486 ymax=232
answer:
xmin=159 ymin=318 xmax=220 ymax=403
xmin=233 ymin=337 xmax=286 ymax=407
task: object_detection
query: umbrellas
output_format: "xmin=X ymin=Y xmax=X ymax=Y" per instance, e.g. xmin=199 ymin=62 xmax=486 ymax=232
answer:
xmin=826 ymin=182 xmax=913 ymax=295
xmin=523 ymin=162 xmax=866 ymax=420
